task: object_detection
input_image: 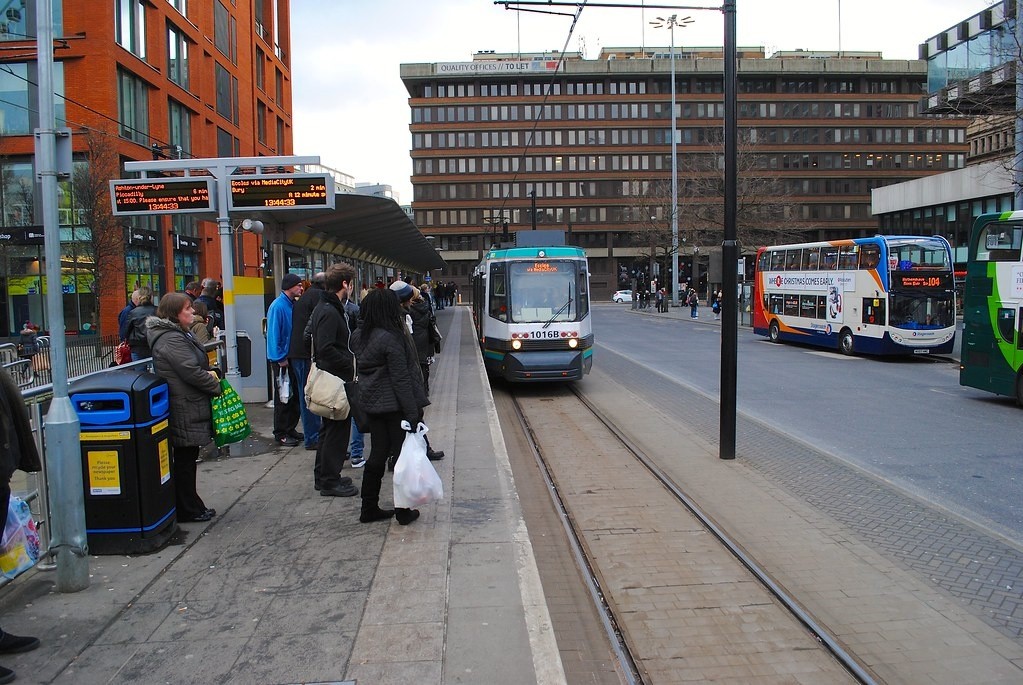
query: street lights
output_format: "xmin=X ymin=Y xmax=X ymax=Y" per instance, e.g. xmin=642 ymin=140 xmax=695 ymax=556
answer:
xmin=649 ymin=14 xmax=695 ymax=307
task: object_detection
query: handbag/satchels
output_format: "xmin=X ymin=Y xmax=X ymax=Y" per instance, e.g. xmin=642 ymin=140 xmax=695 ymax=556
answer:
xmin=210 ymin=379 xmax=250 ymax=448
xmin=32 ymin=353 xmax=49 ymax=371
xmin=304 ymin=362 xmax=350 ymax=420
xmin=344 ymin=379 xmax=383 ymax=433
xmin=17 ymin=344 xmax=24 ymax=357
xmin=713 ymin=302 xmax=717 ymax=307
xmin=0 ymin=493 xmax=41 ymax=579
xmin=276 ymin=367 xmax=293 ymax=404
xmin=116 ymin=341 xmax=131 ymax=365
xmin=393 ymin=420 xmax=443 ymax=509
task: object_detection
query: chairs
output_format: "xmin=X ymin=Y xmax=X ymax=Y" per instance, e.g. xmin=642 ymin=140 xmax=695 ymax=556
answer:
xmin=773 ymin=262 xmax=874 ymax=270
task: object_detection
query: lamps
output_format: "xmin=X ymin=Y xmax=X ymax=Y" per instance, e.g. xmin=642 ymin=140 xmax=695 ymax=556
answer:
xmin=233 ymin=219 xmax=265 ymax=235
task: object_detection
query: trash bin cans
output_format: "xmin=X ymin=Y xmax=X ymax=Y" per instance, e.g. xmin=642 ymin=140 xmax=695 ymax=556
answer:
xmin=69 ymin=370 xmax=179 ymax=556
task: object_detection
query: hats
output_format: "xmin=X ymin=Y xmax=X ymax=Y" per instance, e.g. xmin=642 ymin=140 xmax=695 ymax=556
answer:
xmin=185 ymin=282 xmax=204 ymax=290
xmin=389 ymin=281 xmax=414 ymax=302
xmin=282 ymin=274 xmax=301 ymax=290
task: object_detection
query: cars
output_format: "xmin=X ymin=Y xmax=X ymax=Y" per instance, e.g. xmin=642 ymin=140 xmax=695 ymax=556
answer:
xmin=613 ymin=290 xmax=645 ymax=303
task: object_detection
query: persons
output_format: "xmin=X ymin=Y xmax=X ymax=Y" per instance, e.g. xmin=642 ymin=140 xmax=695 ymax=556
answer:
xmin=656 ymin=288 xmax=666 ymax=313
xmin=266 ymin=263 xmax=444 ymax=525
xmin=434 ymin=281 xmax=458 ymax=310
xmin=147 ymin=293 xmax=222 ymax=524
xmin=681 ymin=288 xmax=699 ymax=320
xmin=118 ymin=278 xmax=224 ymax=373
xmin=0 ymin=364 xmax=40 ymax=685
xmin=19 ymin=320 xmax=40 ymax=378
xmin=638 ymin=290 xmax=651 ymax=309
xmin=713 ymin=288 xmax=722 ymax=320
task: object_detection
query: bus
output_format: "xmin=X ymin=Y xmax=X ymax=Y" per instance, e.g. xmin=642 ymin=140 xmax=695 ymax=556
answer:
xmin=472 ymin=230 xmax=593 ymax=382
xmin=753 ymin=234 xmax=957 ymax=356
xmin=960 ymin=209 xmax=1023 ymax=400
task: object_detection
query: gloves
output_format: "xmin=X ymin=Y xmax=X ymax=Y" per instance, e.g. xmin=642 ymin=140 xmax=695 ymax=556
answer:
xmin=407 ymin=418 xmax=419 ymax=433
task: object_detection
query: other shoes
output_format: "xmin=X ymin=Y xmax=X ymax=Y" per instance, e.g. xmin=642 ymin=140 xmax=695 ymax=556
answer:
xmin=0 ymin=627 xmax=40 ymax=653
xmin=351 ymin=457 xmax=366 ymax=467
xmin=360 ymin=506 xmax=394 ymax=523
xmin=396 ymin=508 xmax=420 ymax=525
xmin=315 ymin=477 xmax=352 ymax=490
xmin=290 ymin=430 xmax=304 ymax=441
xmin=0 ymin=666 xmax=15 ymax=683
xmin=279 ymin=436 xmax=299 ymax=445
xmin=427 ymin=447 xmax=444 ymax=459
xmin=320 ymin=484 xmax=358 ymax=496
